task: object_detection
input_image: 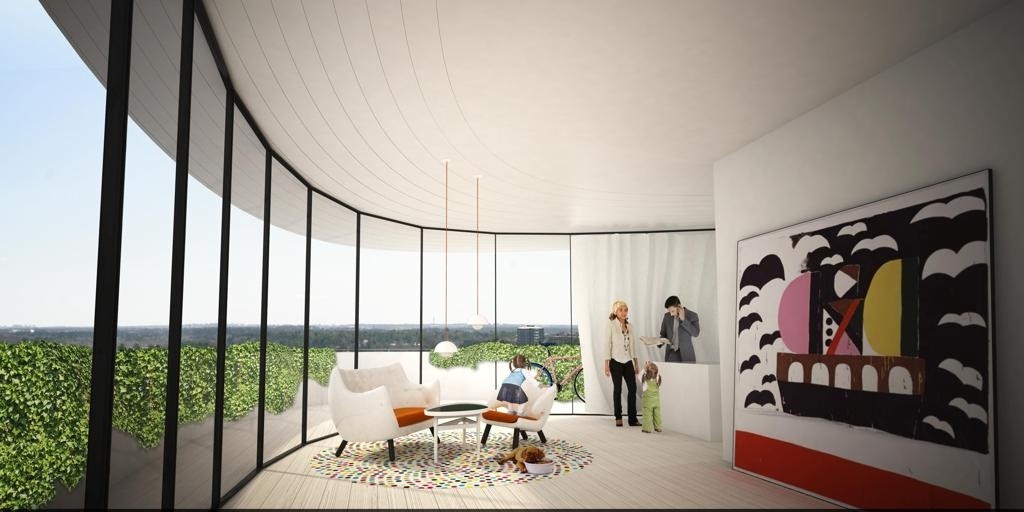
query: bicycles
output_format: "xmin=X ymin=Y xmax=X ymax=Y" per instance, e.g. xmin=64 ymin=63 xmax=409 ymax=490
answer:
xmin=528 ymin=342 xmax=585 ymax=402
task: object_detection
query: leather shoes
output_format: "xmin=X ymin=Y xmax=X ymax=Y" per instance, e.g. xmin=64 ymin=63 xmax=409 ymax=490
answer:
xmin=632 ymin=422 xmax=643 ymax=426
xmin=616 ymin=419 xmax=622 ymax=426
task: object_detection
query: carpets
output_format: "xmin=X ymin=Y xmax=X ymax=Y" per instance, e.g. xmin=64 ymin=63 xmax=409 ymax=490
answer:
xmin=307 ymin=430 xmax=594 ymax=490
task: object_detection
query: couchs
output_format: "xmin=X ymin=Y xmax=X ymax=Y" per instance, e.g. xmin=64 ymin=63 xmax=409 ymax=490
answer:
xmin=481 ymin=385 xmax=556 ymax=450
xmin=328 ymin=362 xmax=440 ymax=462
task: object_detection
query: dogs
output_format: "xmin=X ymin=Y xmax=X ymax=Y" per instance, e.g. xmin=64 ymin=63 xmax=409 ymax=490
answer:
xmin=497 ymin=447 xmax=546 ymax=473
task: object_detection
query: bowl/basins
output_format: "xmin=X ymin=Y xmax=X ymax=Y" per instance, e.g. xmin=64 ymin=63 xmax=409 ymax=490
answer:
xmin=523 ymin=459 xmax=555 ymax=474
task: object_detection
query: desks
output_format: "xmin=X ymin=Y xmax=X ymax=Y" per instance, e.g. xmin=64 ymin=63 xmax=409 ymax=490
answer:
xmin=423 ymin=403 xmax=488 ymax=464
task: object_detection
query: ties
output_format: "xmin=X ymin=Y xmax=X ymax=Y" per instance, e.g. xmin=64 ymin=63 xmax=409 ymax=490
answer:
xmin=673 ymin=317 xmax=679 ymax=352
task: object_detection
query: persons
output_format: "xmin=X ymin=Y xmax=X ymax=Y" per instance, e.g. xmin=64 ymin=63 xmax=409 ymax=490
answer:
xmin=496 ymin=355 xmax=540 ymax=419
xmin=657 ymin=296 xmax=701 ymax=363
xmin=639 ymin=360 xmax=665 ymax=434
xmin=604 ymin=299 xmax=643 ymax=427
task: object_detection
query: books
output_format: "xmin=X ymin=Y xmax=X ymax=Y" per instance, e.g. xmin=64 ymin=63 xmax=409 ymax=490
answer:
xmin=639 ymin=335 xmax=672 ymax=348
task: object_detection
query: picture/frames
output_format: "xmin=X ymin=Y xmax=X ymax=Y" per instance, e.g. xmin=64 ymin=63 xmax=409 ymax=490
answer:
xmin=730 ymin=167 xmax=998 ymax=512
